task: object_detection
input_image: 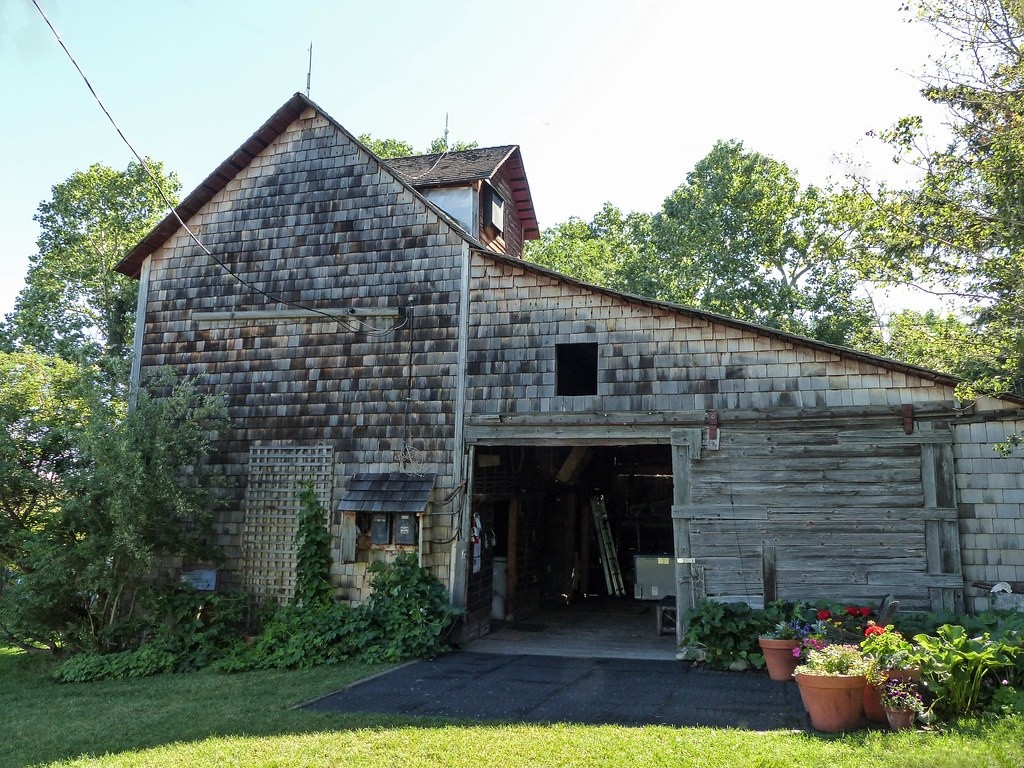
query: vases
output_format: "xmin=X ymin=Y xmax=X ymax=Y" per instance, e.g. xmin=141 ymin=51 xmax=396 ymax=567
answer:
xmin=758 ymin=637 xmax=920 ymax=733
xmin=885 ymin=706 xmax=915 ymax=731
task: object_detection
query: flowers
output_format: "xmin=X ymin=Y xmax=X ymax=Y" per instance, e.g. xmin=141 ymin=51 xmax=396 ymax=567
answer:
xmin=760 ymin=602 xmax=919 ymax=673
xmin=880 ymin=676 xmax=922 ymax=712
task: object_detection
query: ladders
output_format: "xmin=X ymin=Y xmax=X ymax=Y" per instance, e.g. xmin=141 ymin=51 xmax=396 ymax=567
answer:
xmin=590 ymin=489 xmax=626 ymax=597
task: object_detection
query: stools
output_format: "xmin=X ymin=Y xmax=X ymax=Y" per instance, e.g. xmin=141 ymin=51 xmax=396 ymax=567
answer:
xmin=657 ymin=595 xmax=676 ymax=635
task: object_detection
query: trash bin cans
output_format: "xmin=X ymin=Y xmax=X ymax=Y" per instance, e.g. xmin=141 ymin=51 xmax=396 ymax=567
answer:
xmin=491 ymin=557 xmax=509 ymax=621
